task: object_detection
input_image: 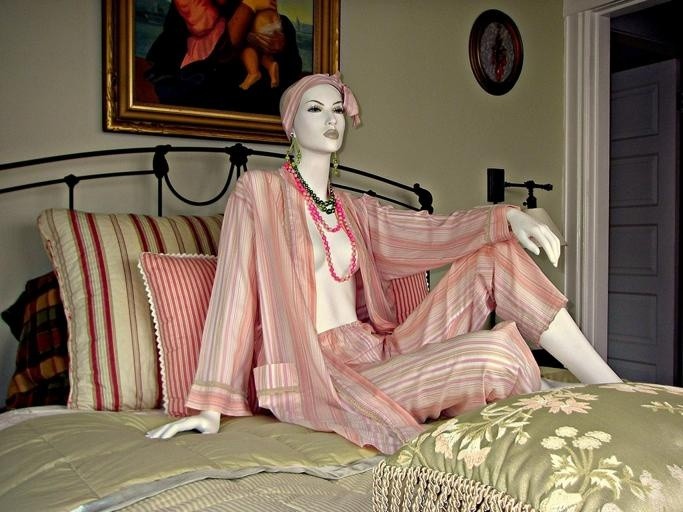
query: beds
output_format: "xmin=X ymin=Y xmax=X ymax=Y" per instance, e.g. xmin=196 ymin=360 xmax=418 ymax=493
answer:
xmin=0 ymin=144 xmax=583 ymax=512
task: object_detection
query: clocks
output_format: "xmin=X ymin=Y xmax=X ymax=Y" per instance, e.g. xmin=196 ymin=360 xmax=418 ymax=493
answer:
xmin=469 ymin=9 xmax=524 ymax=97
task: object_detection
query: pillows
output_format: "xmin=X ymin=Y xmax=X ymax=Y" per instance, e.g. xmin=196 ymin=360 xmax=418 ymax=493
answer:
xmin=373 ymin=382 xmax=683 ymax=512
xmin=137 ymin=251 xmax=263 ymax=417
xmin=389 ymin=272 xmax=429 ymax=324
xmin=37 ymin=208 xmax=224 ymax=410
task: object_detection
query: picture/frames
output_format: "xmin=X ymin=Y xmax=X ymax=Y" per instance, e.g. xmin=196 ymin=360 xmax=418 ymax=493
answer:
xmin=99 ymin=0 xmax=343 ymax=145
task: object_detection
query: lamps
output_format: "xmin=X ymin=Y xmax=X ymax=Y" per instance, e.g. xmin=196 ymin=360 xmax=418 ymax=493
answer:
xmin=485 ymin=167 xmax=568 ymax=249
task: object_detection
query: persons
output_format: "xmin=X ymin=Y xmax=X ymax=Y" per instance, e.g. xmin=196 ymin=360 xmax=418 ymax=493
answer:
xmin=145 ymin=74 xmax=623 ymax=440
xmin=156 ymin=0 xmax=302 ymax=118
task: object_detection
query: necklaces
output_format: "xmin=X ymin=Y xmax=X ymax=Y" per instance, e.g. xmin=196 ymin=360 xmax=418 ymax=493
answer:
xmin=283 ymin=161 xmax=356 ymax=283
xmin=291 ymin=158 xmax=335 ymax=214
